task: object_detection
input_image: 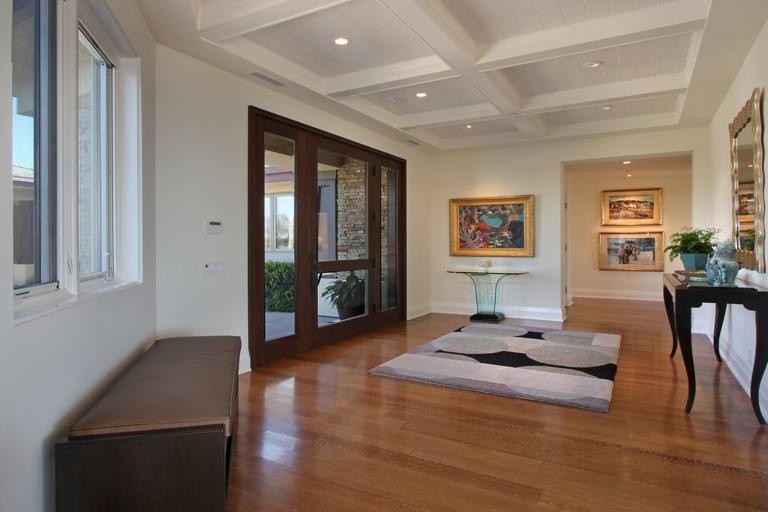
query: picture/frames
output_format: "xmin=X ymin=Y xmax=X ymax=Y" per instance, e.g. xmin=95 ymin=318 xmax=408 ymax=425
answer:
xmin=446 ymin=192 xmax=537 ymax=259
xmin=735 ymin=179 xmax=757 ymax=216
xmin=596 ymin=230 xmax=664 ymax=272
xmin=600 ymin=186 xmax=664 ymax=228
xmin=736 ymin=219 xmax=758 ymax=253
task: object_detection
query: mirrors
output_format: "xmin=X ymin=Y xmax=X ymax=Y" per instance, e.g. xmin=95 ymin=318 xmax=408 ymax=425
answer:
xmin=728 ymin=87 xmax=766 ymax=278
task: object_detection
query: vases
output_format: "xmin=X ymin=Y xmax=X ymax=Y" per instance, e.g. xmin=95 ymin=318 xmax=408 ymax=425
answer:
xmin=704 ymin=256 xmax=740 ymax=284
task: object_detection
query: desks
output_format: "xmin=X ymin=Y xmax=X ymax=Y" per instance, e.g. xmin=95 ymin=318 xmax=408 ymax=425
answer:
xmin=660 ymin=267 xmax=768 ymax=427
xmin=445 ymin=268 xmax=530 ymax=325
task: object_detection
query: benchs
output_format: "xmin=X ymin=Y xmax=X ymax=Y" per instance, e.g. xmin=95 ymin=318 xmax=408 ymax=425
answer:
xmin=52 ymin=334 xmax=244 ymax=511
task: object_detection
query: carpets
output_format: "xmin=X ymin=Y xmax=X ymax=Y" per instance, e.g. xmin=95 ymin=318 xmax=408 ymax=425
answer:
xmin=365 ymin=321 xmax=623 ymax=416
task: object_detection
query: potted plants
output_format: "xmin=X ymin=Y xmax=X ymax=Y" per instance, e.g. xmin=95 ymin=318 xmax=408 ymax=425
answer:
xmin=321 ymin=269 xmax=366 ymax=322
xmin=663 ymin=226 xmax=723 ymax=271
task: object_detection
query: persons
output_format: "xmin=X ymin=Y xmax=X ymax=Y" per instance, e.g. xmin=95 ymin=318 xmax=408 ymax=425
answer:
xmin=618 ymin=244 xmax=638 ymax=264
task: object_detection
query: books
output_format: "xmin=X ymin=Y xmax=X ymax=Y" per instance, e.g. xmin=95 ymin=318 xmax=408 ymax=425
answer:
xmin=674 ymin=270 xmax=709 ymax=282
